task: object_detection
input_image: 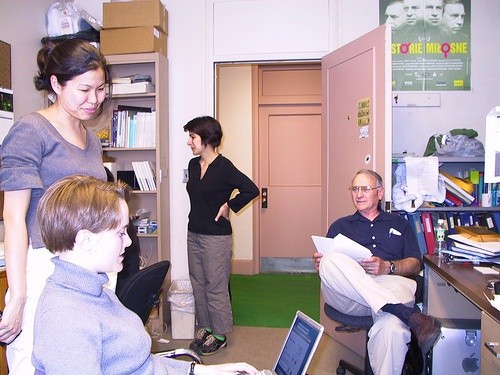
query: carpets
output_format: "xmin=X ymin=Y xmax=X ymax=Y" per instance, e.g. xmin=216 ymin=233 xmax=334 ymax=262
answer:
xmin=229 ymin=275 xmax=321 ymax=327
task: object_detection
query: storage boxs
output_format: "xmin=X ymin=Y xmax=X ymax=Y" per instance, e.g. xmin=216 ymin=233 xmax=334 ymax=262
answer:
xmin=103 ymin=1 xmax=169 ymax=29
xmin=100 ymin=26 xmax=168 ymax=56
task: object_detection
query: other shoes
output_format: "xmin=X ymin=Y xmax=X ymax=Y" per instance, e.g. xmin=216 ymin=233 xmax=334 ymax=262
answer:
xmin=408 ymin=312 xmax=442 ymax=354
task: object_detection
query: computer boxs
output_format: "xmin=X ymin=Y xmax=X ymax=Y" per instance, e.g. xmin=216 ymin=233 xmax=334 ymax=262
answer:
xmin=425 ymin=318 xmax=482 ymax=375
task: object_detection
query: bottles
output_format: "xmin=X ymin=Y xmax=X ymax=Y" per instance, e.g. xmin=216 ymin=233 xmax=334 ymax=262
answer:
xmin=435 ymin=219 xmax=447 ymax=257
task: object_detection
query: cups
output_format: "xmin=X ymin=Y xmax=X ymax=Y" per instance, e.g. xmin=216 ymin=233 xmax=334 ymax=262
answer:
xmin=482 ymin=194 xmax=492 ymax=207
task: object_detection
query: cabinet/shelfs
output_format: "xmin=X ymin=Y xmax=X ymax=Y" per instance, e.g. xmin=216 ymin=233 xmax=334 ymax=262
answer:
xmin=391 ymin=157 xmax=500 ymax=212
xmin=44 ymin=53 xmax=168 ymax=334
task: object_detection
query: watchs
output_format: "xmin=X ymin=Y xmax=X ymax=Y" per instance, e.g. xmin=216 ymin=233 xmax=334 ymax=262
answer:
xmin=387 ymin=260 xmax=397 ymax=274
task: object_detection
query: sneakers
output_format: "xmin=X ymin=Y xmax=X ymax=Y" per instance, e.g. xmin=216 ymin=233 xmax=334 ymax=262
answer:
xmin=190 ymin=327 xmax=213 ymax=349
xmin=198 ymin=334 xmax=227 ymax=356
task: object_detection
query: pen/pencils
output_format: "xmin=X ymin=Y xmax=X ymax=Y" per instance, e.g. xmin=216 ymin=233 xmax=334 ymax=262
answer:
xmin=389 ymin=231 xmax=393 ymax=238
xmin=445 ymin=262 xmax=479 ymax=265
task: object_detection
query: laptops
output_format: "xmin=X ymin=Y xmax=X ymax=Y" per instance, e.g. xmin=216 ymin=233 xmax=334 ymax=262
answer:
xmin=245 ymin=310 xmax=324 ymax=375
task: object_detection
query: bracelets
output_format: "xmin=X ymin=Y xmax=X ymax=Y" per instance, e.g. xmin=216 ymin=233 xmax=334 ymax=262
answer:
xmin=189 ymin=363 xmax=196 ymax=375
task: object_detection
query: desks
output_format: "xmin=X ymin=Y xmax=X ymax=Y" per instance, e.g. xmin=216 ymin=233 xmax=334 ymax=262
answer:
xmin=421 ymin=253 xmax=500 ymax=375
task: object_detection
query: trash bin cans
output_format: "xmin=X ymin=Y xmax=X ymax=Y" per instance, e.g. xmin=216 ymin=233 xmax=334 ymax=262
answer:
xmin=170 ymin=280 xmax=195 ymax=339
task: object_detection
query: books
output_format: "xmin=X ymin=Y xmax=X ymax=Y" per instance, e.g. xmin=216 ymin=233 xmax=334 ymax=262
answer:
xmin=111 ymin=109 xmax=155 ymax=147
xmin=393 ymin=171 xmax=500 ymax=264
xmin=106 ymin=76 xmax=155 ymax=94
xmin=132 ymin=161 xmax=156 ymax=191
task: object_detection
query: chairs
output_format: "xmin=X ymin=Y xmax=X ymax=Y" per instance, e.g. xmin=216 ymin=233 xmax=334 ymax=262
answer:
xmin=118 ymin=260 xmax=173 ymax=327
xmin=324 ymin=303 xmax=424 ymax=375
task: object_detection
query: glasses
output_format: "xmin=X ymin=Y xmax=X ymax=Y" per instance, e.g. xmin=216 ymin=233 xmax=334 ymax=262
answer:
xmin=348 ymin=185 xmax=382 ymax=194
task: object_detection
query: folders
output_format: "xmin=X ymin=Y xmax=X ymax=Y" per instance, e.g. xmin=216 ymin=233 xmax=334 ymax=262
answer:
xmin=478 ymin=213 xmax=496 ymax=232
xmin=430 ymin=212 xmax=443 ymax=250
xmin=421 ymin=213 xmax=436 ymax=254
xmin=407 ymin=213 xmax=427 ymax=254
xmin=440 ymin=212 xmax=481 ymax=249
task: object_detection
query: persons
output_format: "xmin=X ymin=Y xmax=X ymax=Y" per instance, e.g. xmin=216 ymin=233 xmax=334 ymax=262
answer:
xmin=184 ymin=116 xmax=260 ymax=355
xmin=313 ymin=170 xmax=443 ymax=375
xmin=386 ymin=0 xmax=466 ymax=42
xmin=31 ymin=176 xmax=258 ymax=375
xmin=0 ymin=40 xmax=113 ymax=375
